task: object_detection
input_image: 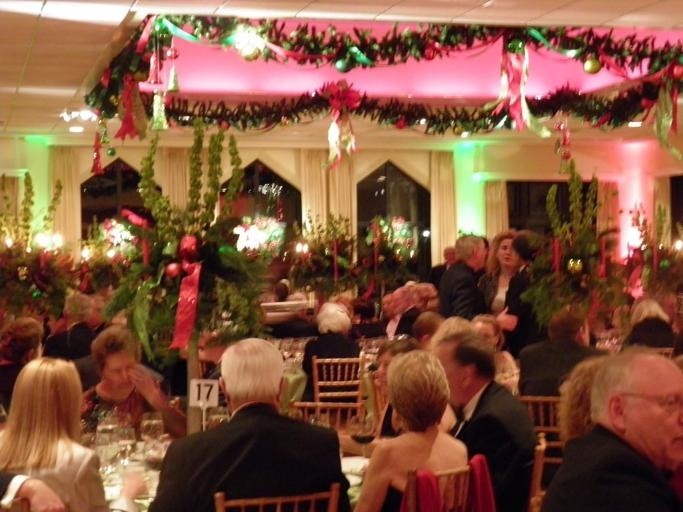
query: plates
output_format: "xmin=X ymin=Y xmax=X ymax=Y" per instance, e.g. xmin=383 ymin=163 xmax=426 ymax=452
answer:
xmin=344 ymin=475 xmax=362 ymax=488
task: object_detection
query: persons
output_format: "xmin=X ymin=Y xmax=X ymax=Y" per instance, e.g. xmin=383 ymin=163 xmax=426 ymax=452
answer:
xmin=0 ymin=226 xmax=683 ymax=512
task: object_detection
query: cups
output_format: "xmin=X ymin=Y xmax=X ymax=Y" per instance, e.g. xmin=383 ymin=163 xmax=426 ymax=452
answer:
xmin=95 ymin=415 xmax=120 ymax=482
xmin=205 ymin=406 xmax=229 ymax=432
xmin=597 ymin=334 xmax=610 ymax=350
xmin=310 ymin=415 xmax=330 ymax=428
xmin=278 ymin=337 xmax=294 ymax=359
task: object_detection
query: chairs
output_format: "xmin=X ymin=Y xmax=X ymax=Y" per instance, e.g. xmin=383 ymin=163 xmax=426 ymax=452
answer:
xmin=0 ymin=321 xmax=682 ymax=511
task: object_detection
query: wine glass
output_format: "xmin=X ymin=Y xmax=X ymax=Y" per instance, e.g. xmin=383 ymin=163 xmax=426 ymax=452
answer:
xmin=138 ymin=411 xmax=165 ymax=475
xmin=348 ymin=414 xmax=377 ymax=465
xmin=116 ymin=427 xmax=137 ymax=468
